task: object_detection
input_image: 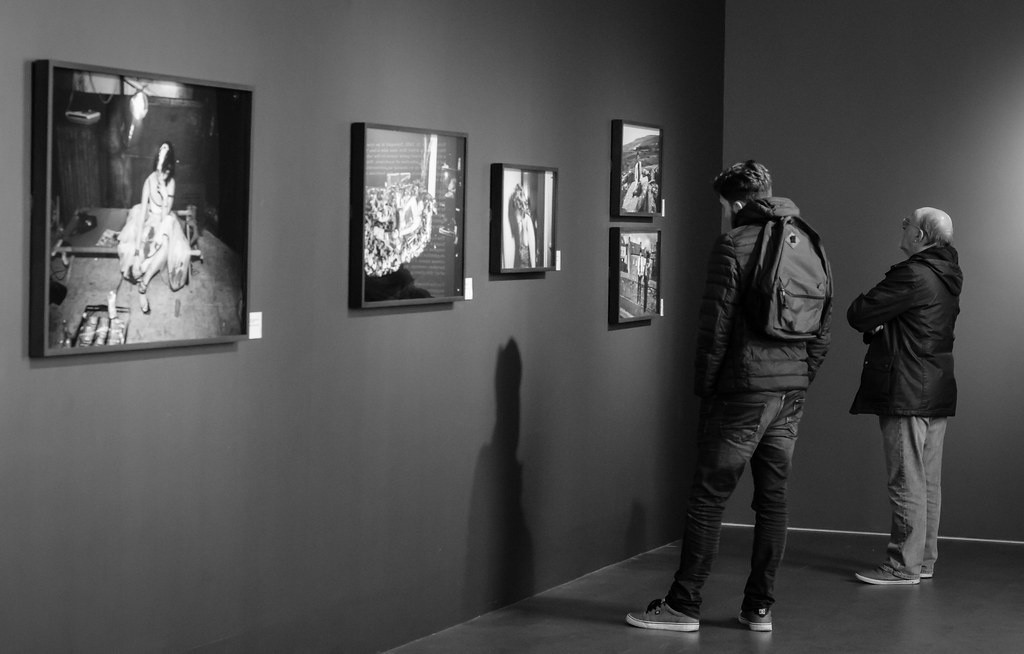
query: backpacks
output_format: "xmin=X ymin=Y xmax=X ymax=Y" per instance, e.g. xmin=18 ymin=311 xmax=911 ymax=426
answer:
xmin=751 ymin=212 xmax=833 ymax=340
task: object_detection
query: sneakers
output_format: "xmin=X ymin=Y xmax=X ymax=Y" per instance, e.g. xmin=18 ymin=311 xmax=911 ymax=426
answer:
xmin=856 ymin=563 xmax=920 ymax=584
xmin=920 ymin=571 xmax=932 ymax=577
xmin=625 ymin=598 xmax=699 ymax=632
xmin=738 ymin=607 xmax=772 ymax=631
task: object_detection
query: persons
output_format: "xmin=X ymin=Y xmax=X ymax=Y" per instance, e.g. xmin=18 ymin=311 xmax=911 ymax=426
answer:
xmin=513 ymin=183 xmax=536 ymax=268
xmin=626 ymin=161 xmax=834 ymax=631
xmin=845 ymin=208 xmax=963 ymax=585
xmin=108 ymin=142 xmax=175 ymax=319
xmin=637 ymin=249 xmax=652 ymax=312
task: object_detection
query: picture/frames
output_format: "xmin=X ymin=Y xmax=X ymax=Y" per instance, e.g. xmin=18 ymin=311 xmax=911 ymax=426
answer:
xmin=29 ymin=60 xmax=253 ymax=358
xmin=611 ymin=118 xmax=663 ymax=221
xmin=490 ymin=163 xmax=558 ymax=274
xmin=609 ymin=228 xmax=661 ymax=329
xmin=349 ymin=122 xmax=467 ymax=306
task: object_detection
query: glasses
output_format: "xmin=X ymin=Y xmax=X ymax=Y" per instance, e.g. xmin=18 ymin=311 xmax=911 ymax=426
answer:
xmin=902 ymin=217 xmax=920 ymax=233
